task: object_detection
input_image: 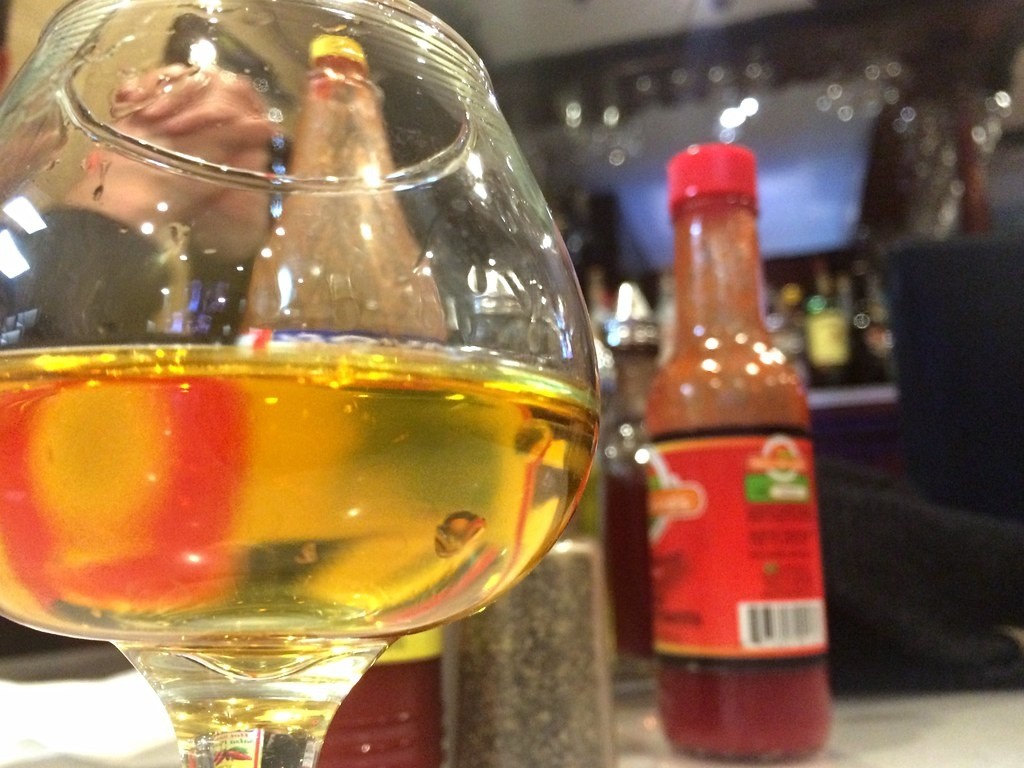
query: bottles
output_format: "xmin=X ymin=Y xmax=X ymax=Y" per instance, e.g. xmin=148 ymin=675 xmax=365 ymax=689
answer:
xmin=775 ymin=243 xmax=902 ymax=390
xmin=246 ymin=37 xmax=445 ymax=768
xmin=600 ymin=283 xmax=668 ymax=681
xmin=640 ymin=145 xmax=833 ymax=762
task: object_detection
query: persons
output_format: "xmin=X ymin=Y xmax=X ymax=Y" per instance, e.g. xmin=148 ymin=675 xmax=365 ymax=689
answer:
xmin=848 ymin=43 xmax=988 ymax=380
xmin=1 ymin=0 xmax=285 ymax=657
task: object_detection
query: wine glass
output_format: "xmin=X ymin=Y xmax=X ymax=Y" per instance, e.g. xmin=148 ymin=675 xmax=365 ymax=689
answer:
xmin=0 ymin=3 xmax=597 ymax=767
